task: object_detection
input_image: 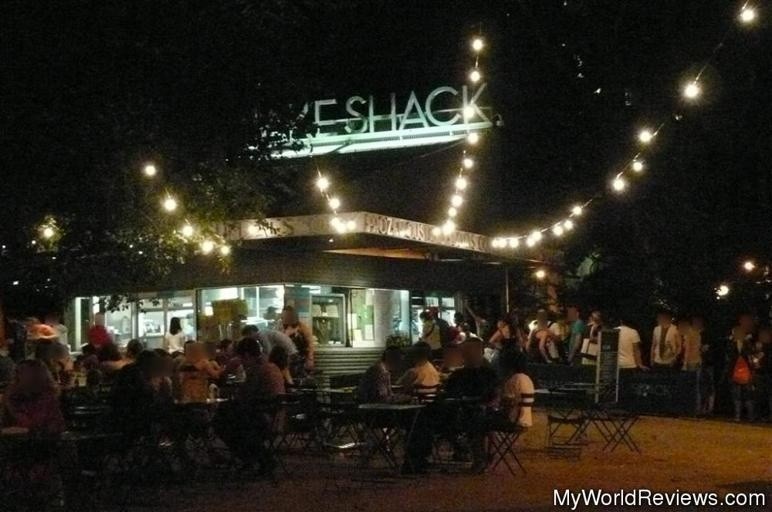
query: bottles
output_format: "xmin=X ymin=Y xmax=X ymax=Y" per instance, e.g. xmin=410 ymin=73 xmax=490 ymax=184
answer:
xmin=208 ymin=381 xmax=218 ymax=402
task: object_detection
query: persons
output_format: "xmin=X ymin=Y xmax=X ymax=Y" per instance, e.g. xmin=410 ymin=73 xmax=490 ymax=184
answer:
xmin=650 ymin=311 xmax=682 ymax=370
xmin=1 ymin=307 xmax=313 ymax=510
xmin=568 ymin=306 xmax=588 ymax=361
xmin=719 ymin=315 xmax=766 ymax=422
xmin=684 ymin=315 xmax=716 ymax=416
xmin=610 ymin=316 xmax=649 ymax=372
xmin=357 ymin=308 xmax=568 ymax=472
xmin=582 ymin=311 xmax=603 ymax=363
